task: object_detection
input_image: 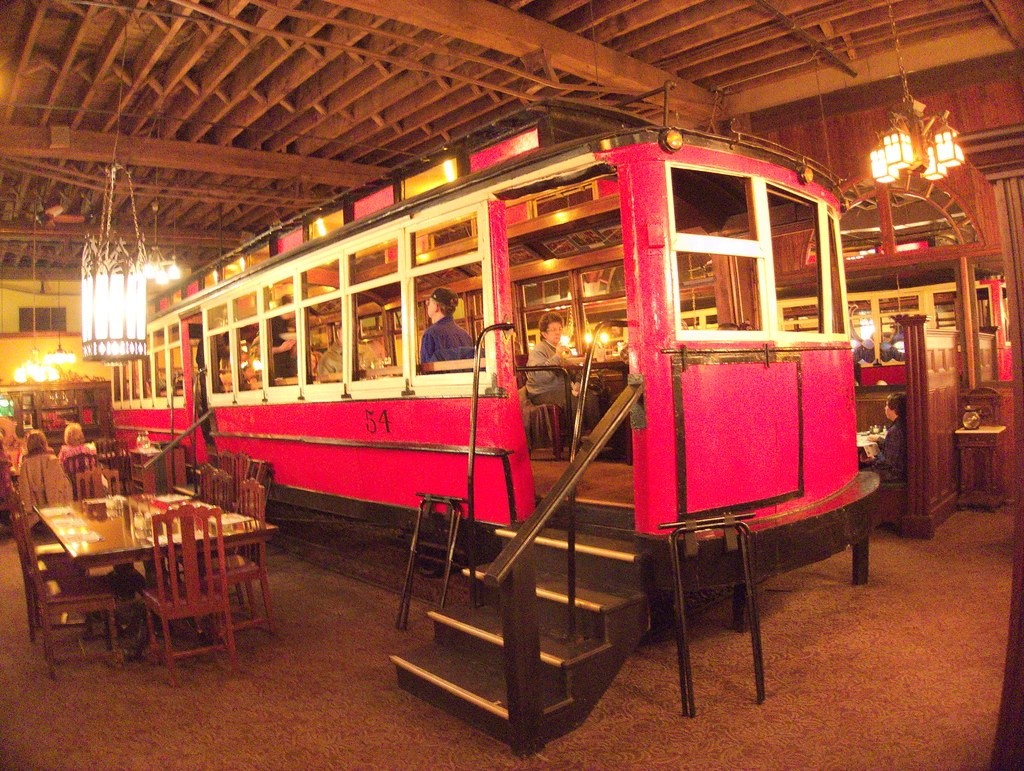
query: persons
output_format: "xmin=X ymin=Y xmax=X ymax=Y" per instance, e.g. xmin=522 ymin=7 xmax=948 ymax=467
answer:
xmin=860 ymin=392 xmax=908 ymax=482
xmin=197 ymin=288 xmax=479 ymax=446
xmin=0 ymin=417 xmax=108 ymax=536
xmin=526 ymin=312 xmax=613 ymax=457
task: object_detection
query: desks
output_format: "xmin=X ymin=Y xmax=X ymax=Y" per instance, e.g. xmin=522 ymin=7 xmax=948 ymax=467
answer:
xmin=32 ymin=493 xmax=279 ymax=638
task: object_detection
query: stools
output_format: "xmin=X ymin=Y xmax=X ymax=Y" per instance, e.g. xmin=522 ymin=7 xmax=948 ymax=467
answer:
xmin=956 ymin=387 xmax=1005 ymax=504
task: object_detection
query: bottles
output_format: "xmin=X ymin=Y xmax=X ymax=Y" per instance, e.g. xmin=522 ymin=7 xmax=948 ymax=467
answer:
xmin=869 ymin=426 xmax=873 ymax=433
xmin=135 ymin=431 xmax=150 ymax=449
xmin=105 ymin=493 xmax=161 ymax=532
xmin=883 ymin=426 xmax=887 ymax=433
xmin=873 ymin=425 xmax=879 ymax=434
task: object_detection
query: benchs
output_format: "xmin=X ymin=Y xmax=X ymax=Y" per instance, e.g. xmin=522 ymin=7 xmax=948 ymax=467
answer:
xmin=867 ymin=479 xmax=905 ymax=530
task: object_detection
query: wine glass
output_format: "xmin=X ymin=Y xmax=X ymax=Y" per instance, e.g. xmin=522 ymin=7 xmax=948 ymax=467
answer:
xmin=42 ymin=390 xmax=72 ymax=405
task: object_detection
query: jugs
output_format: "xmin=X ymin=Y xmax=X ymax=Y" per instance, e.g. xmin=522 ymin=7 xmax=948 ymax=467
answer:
xmin=962 ymin=405 xmax=984 ymax=430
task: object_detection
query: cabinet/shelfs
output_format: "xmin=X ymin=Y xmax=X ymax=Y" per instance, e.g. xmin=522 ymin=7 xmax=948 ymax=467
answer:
xmin=1 ymin=380 xmax=111 ymax=444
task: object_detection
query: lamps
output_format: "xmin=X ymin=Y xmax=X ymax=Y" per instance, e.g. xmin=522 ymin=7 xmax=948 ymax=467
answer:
xmin=81 ymin=16 xmax=146 ymax=366
xmin=869 ymin=1 xmax=964 ymax=183
xmin=44 ymin=298 xmax=78 ymax=369
xmin=12 ymin=176 xmax=59 ymax=382
xmin=137 ymin=119 xmax=179 ymax=283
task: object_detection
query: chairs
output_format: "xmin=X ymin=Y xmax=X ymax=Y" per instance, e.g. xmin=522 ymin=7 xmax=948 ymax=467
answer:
xmin=515 ymin=354 xmax=564 ymax=460
xmin=0 ymin=436 xmax=275 ymax=686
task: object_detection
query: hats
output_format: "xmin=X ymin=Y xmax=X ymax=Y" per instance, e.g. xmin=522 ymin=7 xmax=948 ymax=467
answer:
xmin=431 ymin=288 xmax=460 ymax=307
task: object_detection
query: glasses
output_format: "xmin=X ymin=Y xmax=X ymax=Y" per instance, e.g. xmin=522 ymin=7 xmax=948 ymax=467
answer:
xmin=546 ymin=327 xmax=564 ymax=332
xmin=426 ymin=299 xmax=440 ymax=305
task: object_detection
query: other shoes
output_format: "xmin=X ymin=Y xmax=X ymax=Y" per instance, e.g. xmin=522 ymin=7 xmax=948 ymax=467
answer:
xmin=585 ymin=429 xmax=612 ymax=452
